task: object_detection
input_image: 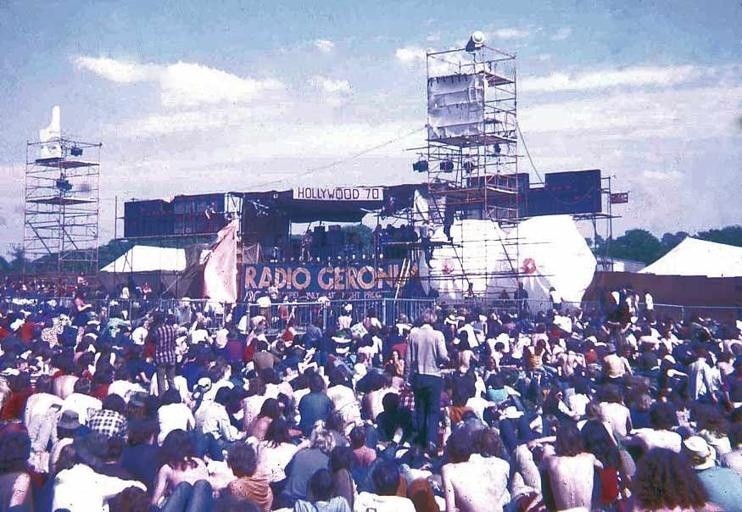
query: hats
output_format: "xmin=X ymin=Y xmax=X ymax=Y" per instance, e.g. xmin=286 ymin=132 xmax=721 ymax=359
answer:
xmin=56 ymin=409 xmax=81 ymax=430
xmin=678 ymin=436 xmax=716 ymax=470
xmin=86 ymin=410 xmax=127 ymax=445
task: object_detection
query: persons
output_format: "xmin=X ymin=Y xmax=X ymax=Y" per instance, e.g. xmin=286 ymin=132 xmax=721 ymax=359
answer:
xmin=442 ymin=153 xmax=451 ymax=162
xmin=418 ymin=153 xmax=427 ymax=160
xmin=1 ymin=274 xmax=742 ymax=512
xmin=204 ymin=202 xmax=215 ymax=219
xmin=372 ymin=207 xmax=456 ymax=263
